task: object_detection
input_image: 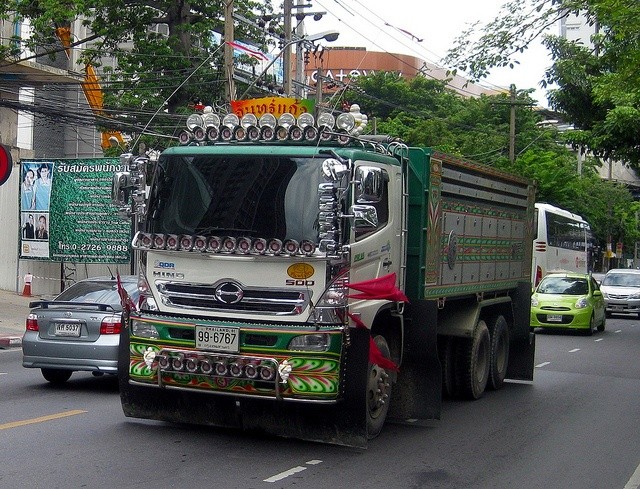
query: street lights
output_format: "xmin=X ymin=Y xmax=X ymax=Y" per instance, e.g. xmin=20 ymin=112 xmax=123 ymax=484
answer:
xmin=236 ymin=30 xmax=344 ymax=100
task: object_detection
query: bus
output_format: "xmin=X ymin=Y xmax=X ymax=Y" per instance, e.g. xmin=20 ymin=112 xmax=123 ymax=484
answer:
xmin=531 ymin=199 xmax=589 ymax=295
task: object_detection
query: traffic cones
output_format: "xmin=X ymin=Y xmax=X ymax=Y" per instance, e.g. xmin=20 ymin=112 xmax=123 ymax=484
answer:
xmin=19 ymin=272 xmax=34 ymax=297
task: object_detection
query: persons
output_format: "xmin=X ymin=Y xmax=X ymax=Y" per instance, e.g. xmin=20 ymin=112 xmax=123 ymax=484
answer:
xmin=35 ymin=216 xmax=48 ymax=240
xmin=31 ymin=164 xmax=51 ymax=210
xmin=21 ymin=169 xmax=35 ymax=210
xmin=22 ymin=214 xmax=34 ymax=239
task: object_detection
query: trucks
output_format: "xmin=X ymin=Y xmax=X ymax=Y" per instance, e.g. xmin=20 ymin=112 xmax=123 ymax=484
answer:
xmin=114 ymin=103 xmax=537 ymax=456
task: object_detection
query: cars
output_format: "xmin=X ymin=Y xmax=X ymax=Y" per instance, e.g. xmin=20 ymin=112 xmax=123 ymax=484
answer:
xmin=531 ymin=270 xmax=606 ymax=337
xmin=600 ymin=268 xmax=639 ymax=317
xmin=21 ymin=276 xmax=139 ymax=391
